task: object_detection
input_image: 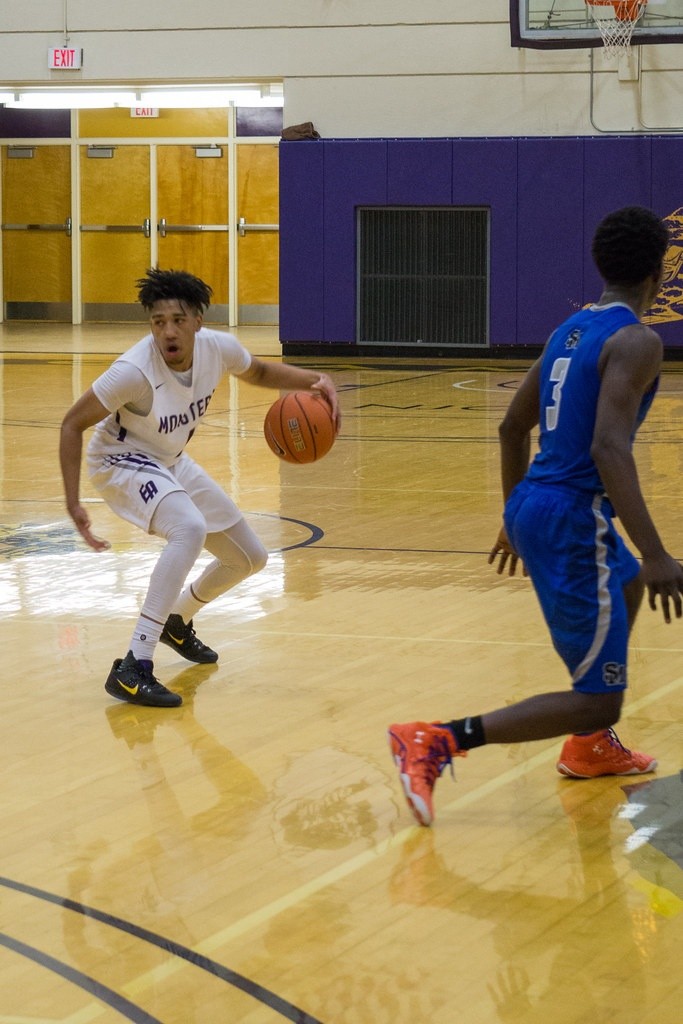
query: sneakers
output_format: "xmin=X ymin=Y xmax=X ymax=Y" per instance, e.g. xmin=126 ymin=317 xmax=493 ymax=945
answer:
xmin=107 ymin=703 xmax=185 ymax=747
xmin=388 ymin=721 xmax=467 ymax=826
xmin=562 ymin=779 xmax=652 ymax=822
xmin=158 ymin=664 xmax=220 ymax=719
xmin=394 ymin=828 xmax=441 ymax=908
xmin=557 ymin=726 xmax=657 ymax=778
xmin=104 ymin=659 xmax=182 ymax=706
xmin=159 ymin=614 xmax=218 ymax=663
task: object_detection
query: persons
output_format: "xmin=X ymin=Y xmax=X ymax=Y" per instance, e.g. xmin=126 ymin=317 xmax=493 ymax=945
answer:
xmin=390 ymin=209 xmax=683 ymax=825
xmin=59 ymin=270 xmax=341 ymax=707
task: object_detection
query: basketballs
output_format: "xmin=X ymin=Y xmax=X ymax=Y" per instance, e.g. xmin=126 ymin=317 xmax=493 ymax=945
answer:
xmin=262 ymin=391 xmax=338 ymax=465
